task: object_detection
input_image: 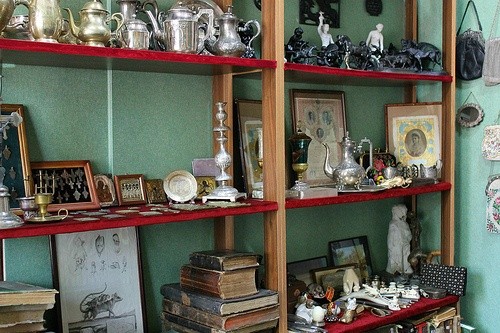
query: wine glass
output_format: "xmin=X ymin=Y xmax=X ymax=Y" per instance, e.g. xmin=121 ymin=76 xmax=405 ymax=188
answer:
xmin=32 ymin=193 xmax=51 ymax=221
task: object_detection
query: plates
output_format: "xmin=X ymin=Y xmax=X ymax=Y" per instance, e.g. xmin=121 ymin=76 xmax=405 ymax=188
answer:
xmin=163 ymin=171 xmax=198 ymax=202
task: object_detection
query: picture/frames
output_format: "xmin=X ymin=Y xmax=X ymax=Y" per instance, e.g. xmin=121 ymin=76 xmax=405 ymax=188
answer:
xmin=49 ymin=226 xmax=149 ymax=333
xmin=289 ymin=88 xmax=347 ymax=188
xmin=114 ymin=174 xmax=147 ymax=206
xmin=328 ymin=235 xmax=374 ymax=284
xmin=384 ymin=102 xmax=443 ymax=181
xmin=234 ymin=98 xmax=290 ymax=199
xmin=311 ymin=263 xmax=358 ymax=285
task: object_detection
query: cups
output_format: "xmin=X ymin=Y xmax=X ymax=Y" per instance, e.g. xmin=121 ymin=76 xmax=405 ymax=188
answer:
xmin=420 ymin=164 xmax=437 ymax=178
xmin=5 ymin=15 xmax=29 ymax=37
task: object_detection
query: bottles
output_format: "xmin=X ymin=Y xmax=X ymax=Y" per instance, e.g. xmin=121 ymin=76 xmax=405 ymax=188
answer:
xmin=0 ymin=183 xmax=25 ymax=229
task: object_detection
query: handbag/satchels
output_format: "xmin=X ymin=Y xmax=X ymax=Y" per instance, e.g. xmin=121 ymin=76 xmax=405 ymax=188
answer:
xmin=485 ymin=173 xmax=500 ymax=234
xmin=482 ymin=112 xmax=500 ymax=161
xmin=482 ymin=0 xmax=500 ymax=87
xmin=456 ymin=0 xmax=485 ymax=81
xmin=355 ymin=147 xmax=396 ymax=185
xmin=420 ymin=263 xmax=467 ymax=297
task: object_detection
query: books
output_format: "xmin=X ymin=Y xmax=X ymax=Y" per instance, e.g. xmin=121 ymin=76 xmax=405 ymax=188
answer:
xmin=408 ymin=307 xmax=461 ymax=333
xmin=0 ymin=281 xmax=59 ymax=333
xmin=160 ymin=248 xmax=281 ymax=333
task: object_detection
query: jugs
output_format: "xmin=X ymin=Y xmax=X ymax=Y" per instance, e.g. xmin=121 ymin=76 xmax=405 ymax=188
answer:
xmin=382 ymin=159 xmax=397 ymax=179
xmin=14 ymin=0 xmax=72 ymax=43
xmin=0 ymin=0 xmax=21 ymax=37
xmin=111 ymin=0 xmax=159 ymax=49
xmin=200 ymin=4 xmax=261 ymax=58
xmin=63 ymin=0 xmax=124 ymax=47
xmin=113 ymin=14 xmax=154 ymax=51
xmin=318 ymin=130 xmax=373 ymax=191
xmin=145 ymin=2 xmax=214 ymax=54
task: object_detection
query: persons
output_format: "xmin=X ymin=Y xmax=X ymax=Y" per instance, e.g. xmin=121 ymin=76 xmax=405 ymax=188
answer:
xmin=306 ymin=109 xmax=333 ymax=138
xmin=408 ymin=131 xmax=426 ymax=157
xmin=317 ymin=21 xmax=335 ymax=67
xmin=285 ymin=27 xmax=305 ymax=62
xmin=365 ymin=23 xmax=384 ymax=67
xmin=121 ymin=183 xmax=141 ymax=198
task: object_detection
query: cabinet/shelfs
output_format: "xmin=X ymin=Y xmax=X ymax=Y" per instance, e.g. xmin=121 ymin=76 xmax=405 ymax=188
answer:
xmin=0 ymin=0 xmax=462 ymax=333
